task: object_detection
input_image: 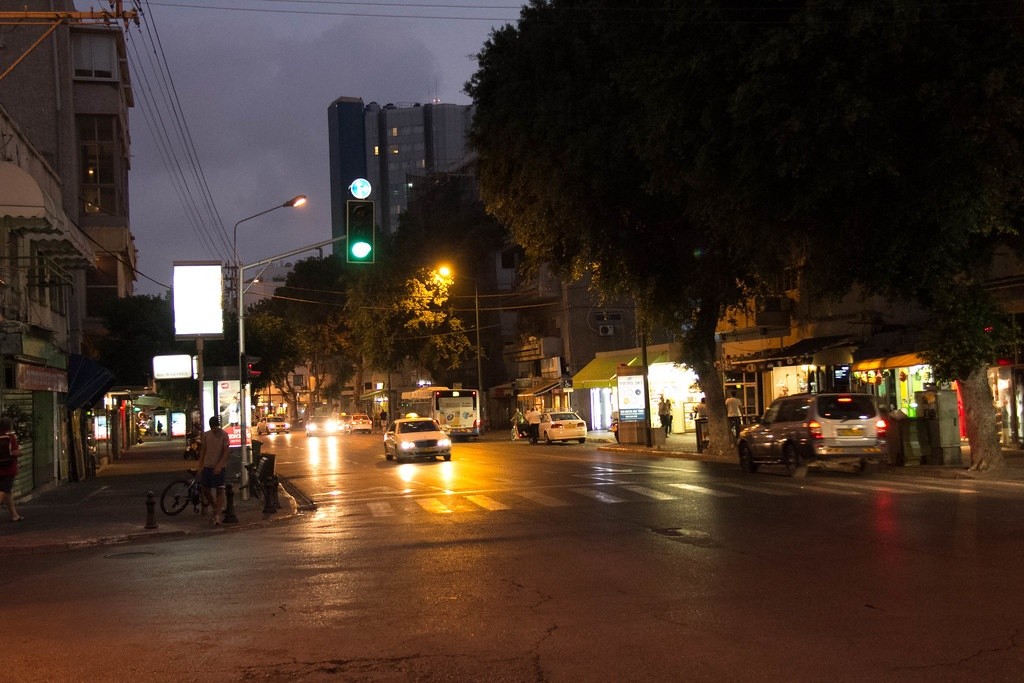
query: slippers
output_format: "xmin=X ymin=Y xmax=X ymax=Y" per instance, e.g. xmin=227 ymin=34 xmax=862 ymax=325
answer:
xmin=10 ymin=516 xmax=24 ymax=521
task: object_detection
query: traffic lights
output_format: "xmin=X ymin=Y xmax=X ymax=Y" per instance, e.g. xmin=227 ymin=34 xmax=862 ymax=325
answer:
xmin=346 ymin=200 xmax=376 ymax=263
xmin=242 ymin=354 xmax=261 ymax=385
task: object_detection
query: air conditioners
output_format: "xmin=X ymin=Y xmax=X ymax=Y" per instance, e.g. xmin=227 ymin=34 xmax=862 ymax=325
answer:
xmin=599 ymin=325 xmax=613 ymax=335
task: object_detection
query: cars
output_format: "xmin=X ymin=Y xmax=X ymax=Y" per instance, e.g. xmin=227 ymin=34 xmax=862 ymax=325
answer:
xmin=538 ymin=412 xmax=587 ymax=444
xmin=383 ymin=412 xmax=452 ymax=463
xmin=305 ymin=412 xmax=373 ymax=436
xmin=257 ymin=417 xmax=290 ymax=435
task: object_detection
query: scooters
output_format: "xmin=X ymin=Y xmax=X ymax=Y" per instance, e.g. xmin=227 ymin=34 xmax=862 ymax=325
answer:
xmin=610 ymin=416 xmax=621 ymax=444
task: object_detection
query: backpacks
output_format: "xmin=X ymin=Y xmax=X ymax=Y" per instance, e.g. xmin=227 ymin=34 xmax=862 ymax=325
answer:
xmin=0 ymin=435 xmax=13 ymax=464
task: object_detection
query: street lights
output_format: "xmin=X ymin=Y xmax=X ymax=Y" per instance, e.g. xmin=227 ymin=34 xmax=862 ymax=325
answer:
xmin=439 ymin=266 xmax=486 ymax=435
xmin=232 ymin=195 xmax=309 ymax=265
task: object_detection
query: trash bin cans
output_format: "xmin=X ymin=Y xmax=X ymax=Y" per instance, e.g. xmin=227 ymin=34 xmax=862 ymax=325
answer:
xmin=695 ymin=417 xmax=709 ymax=453
xmin=252 ymin=439 xmax=264 ymax=465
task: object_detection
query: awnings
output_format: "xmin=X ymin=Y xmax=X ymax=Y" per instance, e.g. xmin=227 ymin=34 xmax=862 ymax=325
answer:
xmin=732 ymin=340 xmax=853 ymax=364
xmin=572 ymin=352 xmax=661 ymax=388
xmin=351 ymin=390 xmax=390 ymax=400
xmin=517 ymin=381 xmax=560 ymax=397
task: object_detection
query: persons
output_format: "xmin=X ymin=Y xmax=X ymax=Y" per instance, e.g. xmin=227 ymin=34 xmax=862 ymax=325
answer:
xmin=698 ymin=397 xmax=707 ymax=440
xmin=380 ymin=411 xmax=387 ymax=425
xmin=158 ymin=421 xmax=163 ymax=437
xmin=199 ymin=417 xmax=231 ymax=525
xmin=221 ymin=392 xmax=242 ymax=426
xmin=0 ymin=417 xmax=24 ymax=520
xmin=726 ymin=390 xmax=743 ymax=437
xmin=658 ymin=397 xmax=673 ymax=438
xmin=511 ymin=407 xmax=541 ymax=444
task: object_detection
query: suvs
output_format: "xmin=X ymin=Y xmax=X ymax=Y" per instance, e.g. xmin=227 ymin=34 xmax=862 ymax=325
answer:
xmin=735 ymin=390 xmax=888 ymax=478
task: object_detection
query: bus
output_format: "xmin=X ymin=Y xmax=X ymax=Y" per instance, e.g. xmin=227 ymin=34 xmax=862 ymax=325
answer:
xmin=401 ymin=387 xmax=480 ymax=440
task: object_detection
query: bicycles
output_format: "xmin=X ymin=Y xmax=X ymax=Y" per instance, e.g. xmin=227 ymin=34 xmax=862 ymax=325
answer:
xmin=159 ymin=468 xmax=212 ymax=515
xmin=239 ymin=447 xmax=267 ymax=499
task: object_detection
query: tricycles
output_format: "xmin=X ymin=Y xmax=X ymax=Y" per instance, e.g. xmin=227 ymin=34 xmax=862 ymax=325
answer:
xmin=510 ymin=420 xmax=530 ymax=441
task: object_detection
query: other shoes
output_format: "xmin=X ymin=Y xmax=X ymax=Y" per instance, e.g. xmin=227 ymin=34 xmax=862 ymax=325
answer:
xmin=213 ymin=514 xmax=224 ymax=524
